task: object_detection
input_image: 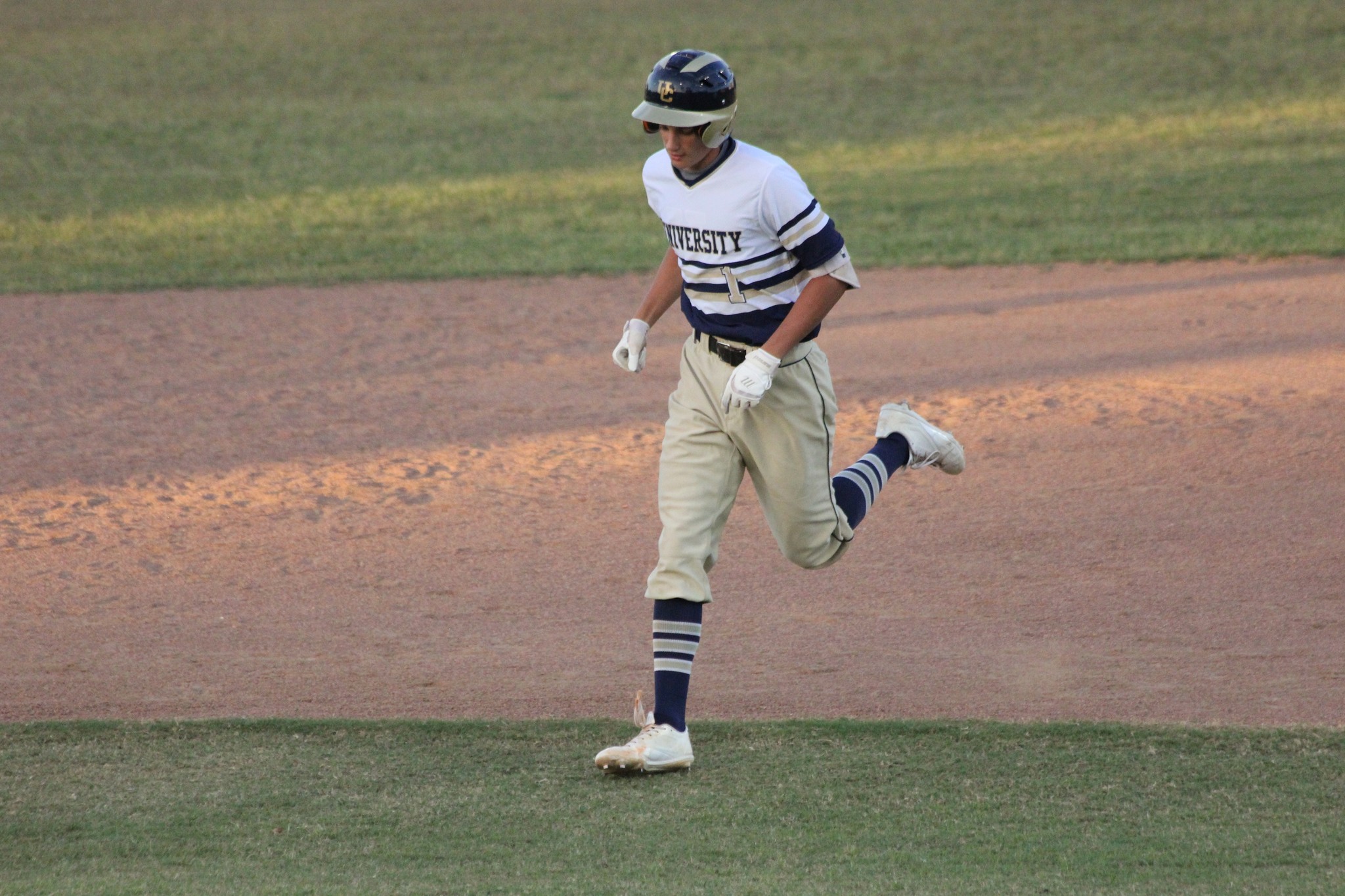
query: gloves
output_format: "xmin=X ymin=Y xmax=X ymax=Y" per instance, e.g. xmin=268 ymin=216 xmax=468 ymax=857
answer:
xmin=611 ymin=318 xmax=650 ymax=373
xmin=720 ymin=349 xmax=782 ymax=414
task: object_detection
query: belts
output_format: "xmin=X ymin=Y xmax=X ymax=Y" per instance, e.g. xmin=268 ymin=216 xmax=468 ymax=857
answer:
xmin=694 ymin=329 xmax=745 ymax=368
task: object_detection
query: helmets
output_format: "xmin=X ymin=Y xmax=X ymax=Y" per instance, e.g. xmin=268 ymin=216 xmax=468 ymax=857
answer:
xmin=632 ymin=48 xmax=737 ymax=148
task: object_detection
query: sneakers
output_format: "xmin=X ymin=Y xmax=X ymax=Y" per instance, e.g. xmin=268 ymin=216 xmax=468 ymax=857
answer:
xmin=595 ymin=688 xmax=694 ymax=771
xmin=876 ymin=403 xmax=965 ymax=474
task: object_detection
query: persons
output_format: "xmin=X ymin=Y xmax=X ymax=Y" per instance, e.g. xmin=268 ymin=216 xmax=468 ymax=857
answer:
xmin=594 ymin=50 xmax=965 ymax=772
